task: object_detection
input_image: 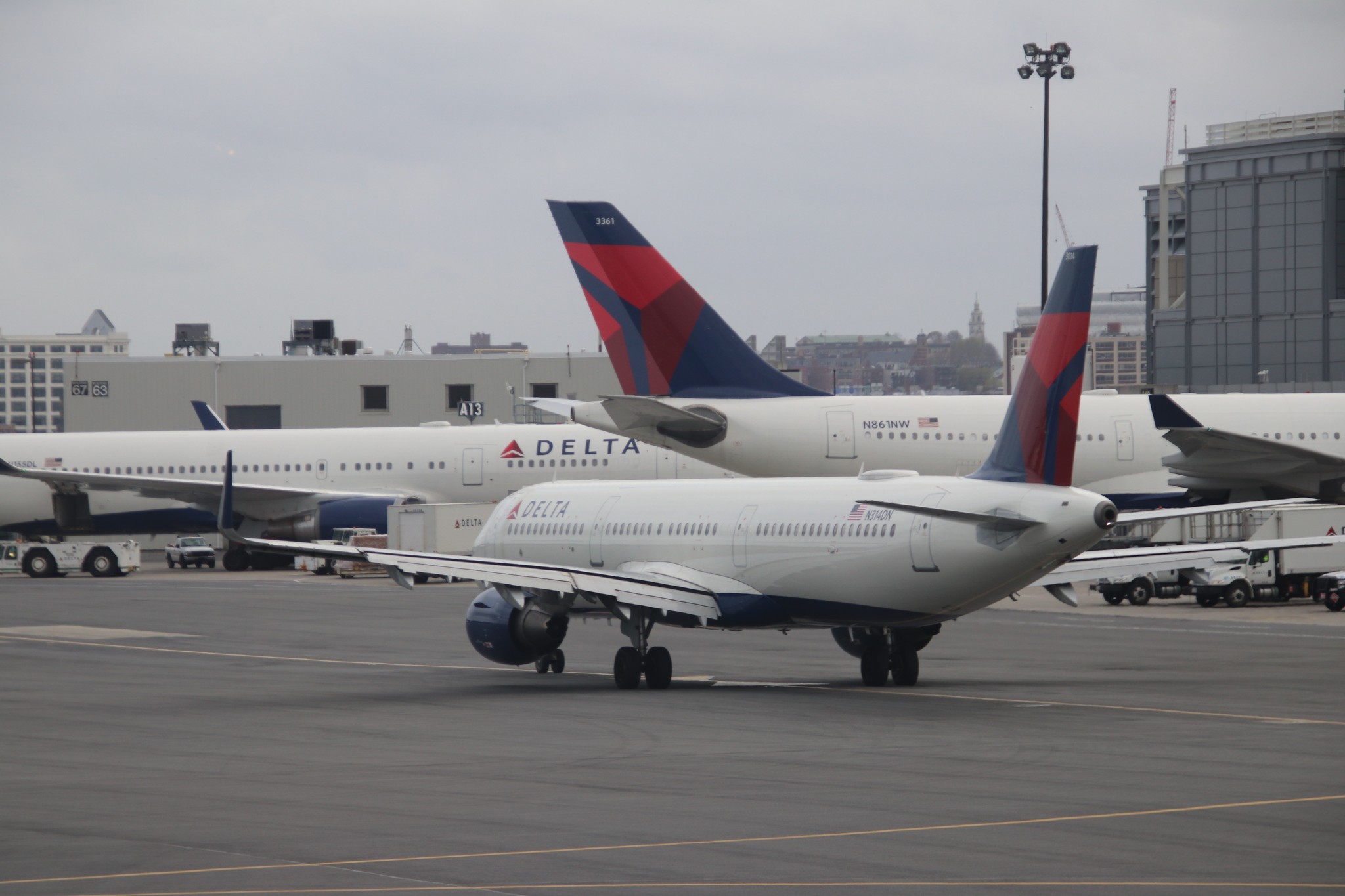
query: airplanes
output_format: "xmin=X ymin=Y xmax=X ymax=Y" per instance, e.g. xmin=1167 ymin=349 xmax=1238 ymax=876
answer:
xmin=234 ymin=243 xmax=1120 ymax=693
xmin=0 ymin=198 xmax=1345 ymax=617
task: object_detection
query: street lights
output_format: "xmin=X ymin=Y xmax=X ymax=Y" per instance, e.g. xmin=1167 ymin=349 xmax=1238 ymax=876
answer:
xmin=1015 ymin=40 xmax=1074 ymax=312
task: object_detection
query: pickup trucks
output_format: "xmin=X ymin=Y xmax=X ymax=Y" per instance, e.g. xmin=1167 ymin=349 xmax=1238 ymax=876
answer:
xmin=164 ymin=536 xmax=216 ymax=570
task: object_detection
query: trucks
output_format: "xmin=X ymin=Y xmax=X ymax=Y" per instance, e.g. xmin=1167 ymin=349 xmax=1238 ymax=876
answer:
xmin=1194 ymin=510 xmax=1345 ymax=608
